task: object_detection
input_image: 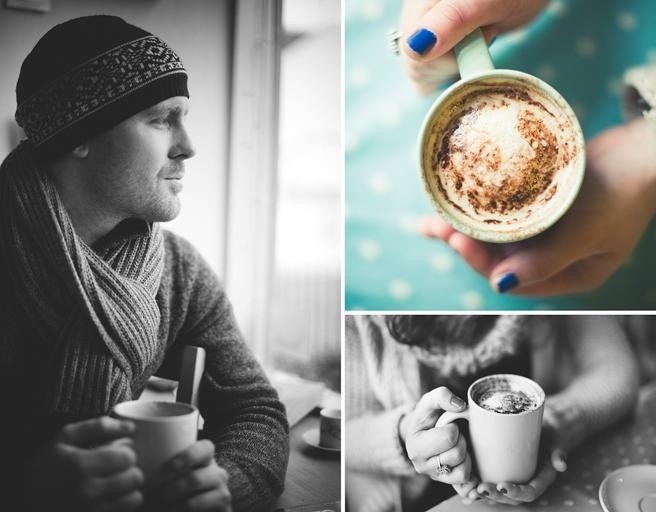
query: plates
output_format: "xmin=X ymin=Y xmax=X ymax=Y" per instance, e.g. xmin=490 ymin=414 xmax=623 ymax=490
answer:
xmin=302 ymin=429 xmax=341 ymax=452
xmin=598 ymin=465 xmax=654 ymax=511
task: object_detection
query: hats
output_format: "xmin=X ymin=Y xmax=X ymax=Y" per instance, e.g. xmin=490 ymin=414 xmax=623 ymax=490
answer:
xmin=15 ymin=15 xmax=189 ymax=160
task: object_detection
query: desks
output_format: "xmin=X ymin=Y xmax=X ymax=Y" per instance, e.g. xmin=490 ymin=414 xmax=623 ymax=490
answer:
xmin=421 ymin=390 xmax=655 ymax=512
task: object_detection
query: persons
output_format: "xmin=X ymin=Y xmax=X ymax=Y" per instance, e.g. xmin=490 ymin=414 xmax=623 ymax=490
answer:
xmin=346 ymin=315 xmax=639 ymax=512
xmin=402 ymin=0 xmax=655 ymax=298
xmin=0 ymin=13 xmax=290 ymax=511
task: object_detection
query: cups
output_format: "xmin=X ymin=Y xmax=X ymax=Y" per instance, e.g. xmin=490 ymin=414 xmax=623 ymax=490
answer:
xmin=114 ymin=401 xmax=199 ymax=494
xmin=421 ymin=29 xmax=585 ymax=244
xmin=438 ymin=374 xmax=546 ymax=485
xmin=319 ymin=410 xmax=341 ymax=446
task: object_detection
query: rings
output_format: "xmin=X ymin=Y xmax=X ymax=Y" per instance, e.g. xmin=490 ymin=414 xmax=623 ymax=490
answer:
xmin=388 ymin=26 xmax=402 ymax=55
xmin=434 ymin=452 xmax=452 ymax=475
